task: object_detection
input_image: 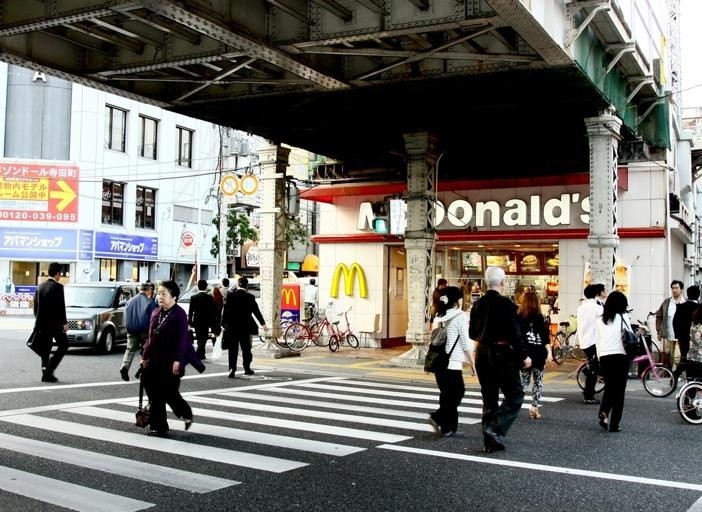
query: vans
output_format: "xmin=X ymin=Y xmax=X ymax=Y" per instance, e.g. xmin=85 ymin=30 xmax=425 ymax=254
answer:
xmin=170 ymin=277 xmax=261 ymax=340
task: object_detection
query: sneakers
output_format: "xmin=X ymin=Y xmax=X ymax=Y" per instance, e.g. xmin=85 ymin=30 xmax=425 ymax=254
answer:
xmin=184 ymin=418 xmax=193 ymax=430
xmin=42 ymin=374 xmax=58 ymax=382
xmin=583 ymin=398 xmax=600 ymax=404
xmin=610 ymin=424 xmax=621 ymax=432
xmin=598 ymin=411 xmax=609 ymax=432
xmin=481 ymin=426 xmax=507 ymax=452
xmin=147 ymin=428 xmax=170 ymax=437
xmin=120 ymin=366 xmax=129 ymax=382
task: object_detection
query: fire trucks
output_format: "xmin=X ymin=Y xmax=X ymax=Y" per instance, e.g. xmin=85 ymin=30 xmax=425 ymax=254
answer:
xmin=637 ymin=311 xmax=664 ymax=365
xmin=574 ymin=320 xmax=678 ymax=397
xmin=552 ymin=319 xmax=588 ymax=365
xmin=326 ymin=306 xmax=360 ymax=352
xmin=674 ymin=371 xmax=702 ymax=425
xmin=259 ymin=301 xmax=336 ymax=352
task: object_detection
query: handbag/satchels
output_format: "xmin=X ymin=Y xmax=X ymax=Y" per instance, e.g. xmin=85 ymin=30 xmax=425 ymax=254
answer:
xmin=619 ymin=312 xmax=640 ymax=357
xmin=25 ymin=326 xmax=42 ymax=356
xmin=134 ymin=364 xmax=153 ymax=429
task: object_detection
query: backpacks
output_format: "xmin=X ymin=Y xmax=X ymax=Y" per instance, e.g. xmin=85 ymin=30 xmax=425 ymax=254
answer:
xmin=424 ymin=311 xmax=464 ymax=373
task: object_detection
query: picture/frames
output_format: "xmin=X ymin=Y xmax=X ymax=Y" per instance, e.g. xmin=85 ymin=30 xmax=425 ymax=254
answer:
xmin=394 ymin=265 xmax=405 ymax=297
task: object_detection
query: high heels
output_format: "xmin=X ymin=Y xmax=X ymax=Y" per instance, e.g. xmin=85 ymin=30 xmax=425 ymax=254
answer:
xmin=528 ymin=408 xmax=543 ymax=419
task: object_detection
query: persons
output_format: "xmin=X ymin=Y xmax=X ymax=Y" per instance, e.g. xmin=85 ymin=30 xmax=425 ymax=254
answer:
xmin=595 ymin=284 xmax=607 ymax=307
xmin=655 ymin=280 xmax=688 ymax=372
xmin=594 ymin=291 xmax=635 ymax=432
xmin=33 ymin=263 xmax=69 ymax=382
xmin=118 ymin=273 xmax=268 ymax=436
xmin=685 ymin=303 xmax=702 ymax=420
xmin=428 ymin=266 xmax=558 ymax=452
xmin=670 ymin=285 xmax=702 ymax=387
xmin=576 ymin=284 xmax=604 ymax=405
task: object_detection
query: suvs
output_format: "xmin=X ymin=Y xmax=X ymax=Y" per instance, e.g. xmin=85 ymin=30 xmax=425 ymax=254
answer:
xmin=51 ymin=281 xmax=145 ymax=354
